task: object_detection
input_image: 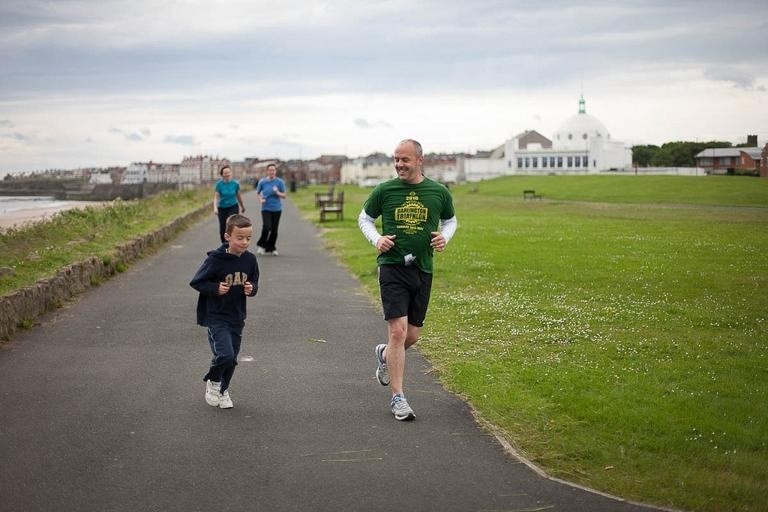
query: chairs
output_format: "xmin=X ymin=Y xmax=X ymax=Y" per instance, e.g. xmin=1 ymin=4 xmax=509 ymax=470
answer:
xmin=314 ymin=181 xmax=345 ymax=221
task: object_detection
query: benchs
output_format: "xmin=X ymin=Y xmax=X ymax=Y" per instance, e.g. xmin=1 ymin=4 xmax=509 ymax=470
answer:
xmin=524 ymin=190 xmax=542 ymax=201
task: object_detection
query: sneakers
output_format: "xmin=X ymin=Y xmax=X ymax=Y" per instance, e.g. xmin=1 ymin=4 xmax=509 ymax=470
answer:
xmin=375 ymin=344 xmax=390 ymax=386
xmin=257 ymin=247 xmax=278 ymax=256
xmin=205 ymin=378 xmax=234 ymax=409
xmin=391 ymin=394 xmax=416 ymax=420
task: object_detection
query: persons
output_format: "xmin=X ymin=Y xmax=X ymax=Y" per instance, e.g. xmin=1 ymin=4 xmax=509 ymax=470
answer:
xmin=190 ymin=214 xmax=259 ymax=410
xmin=358 ymin=139 xmax=458 ymax=422
xmin=214 ymin=165 xmax=246 ymax=244
xmin=255 ymin=163 xmax=287 ymax=256
